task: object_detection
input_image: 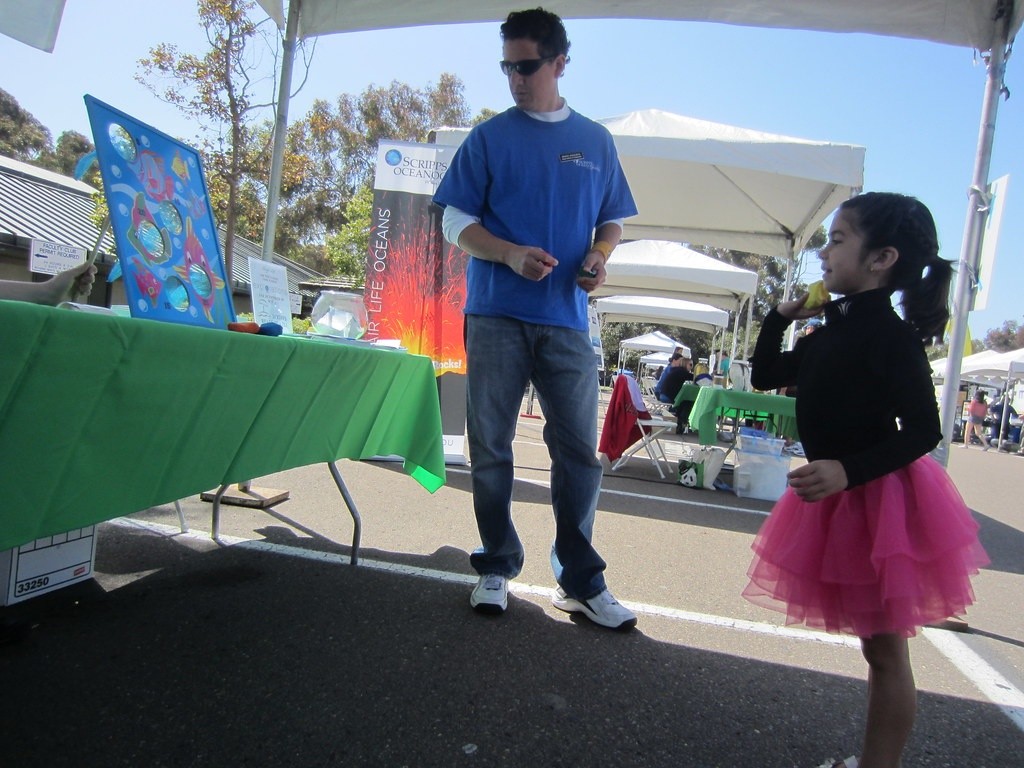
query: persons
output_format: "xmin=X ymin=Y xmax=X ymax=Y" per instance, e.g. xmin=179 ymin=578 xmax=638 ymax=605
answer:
xmin=0 ymin=262 xmax=97 ymax=307
xmin=991 ymin=396 xmax=1018 ymax=440
xmin=655 ymin=347 xmax=694 ymax=435
xmin=721 ymin=352 xmax=729 ymax=378
xmin=959 ymin=389 xmax=990 ymax=451
xmin=432 ymin=10 xmax=638 ymax=631
xmin=655 ymin=366 xmax=664 ymax=380
xmin=751 ymin=192 xmax=992 ymax=768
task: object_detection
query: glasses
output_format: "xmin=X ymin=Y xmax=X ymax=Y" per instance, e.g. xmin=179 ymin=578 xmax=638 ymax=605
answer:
xmin=687 ymin=362 xmax=693 ymax=367
xmin=499 ymin=57 xmax=552 ymax=77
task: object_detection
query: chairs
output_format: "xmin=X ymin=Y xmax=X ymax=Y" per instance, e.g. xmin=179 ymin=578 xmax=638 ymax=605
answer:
xmin=606 ymin=374 xmax=676 ymax=480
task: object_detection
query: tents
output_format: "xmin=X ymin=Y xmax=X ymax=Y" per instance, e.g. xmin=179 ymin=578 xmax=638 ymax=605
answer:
xmin=596 ymin=108 xmax=866 ymax=302
xmin=929 ymin=347 xmax=1024 ymax=453
xmin=588 ymin=240 xmax=758 ymax=360
xmin=616 ymin=331 xmax=691 ymax=378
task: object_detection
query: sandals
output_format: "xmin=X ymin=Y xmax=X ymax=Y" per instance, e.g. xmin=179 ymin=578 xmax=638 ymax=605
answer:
xmin=815 ymin=756 xmax=859 ymax=768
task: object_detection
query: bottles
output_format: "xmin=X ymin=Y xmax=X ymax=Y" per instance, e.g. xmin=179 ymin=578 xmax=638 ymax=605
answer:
xmin=310 ymin=290 xmax=369 ymax=340
xmin=693 ymin=357 xmax=709 ymax=381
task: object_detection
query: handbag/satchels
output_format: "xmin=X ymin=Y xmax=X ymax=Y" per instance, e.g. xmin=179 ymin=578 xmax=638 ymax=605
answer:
xmin=676 ymin=447 xmax=726 ymax=490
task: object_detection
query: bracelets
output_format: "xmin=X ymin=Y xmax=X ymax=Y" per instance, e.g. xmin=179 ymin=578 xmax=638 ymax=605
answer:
xmin=591 ymin=240 xmax=612 ymax=264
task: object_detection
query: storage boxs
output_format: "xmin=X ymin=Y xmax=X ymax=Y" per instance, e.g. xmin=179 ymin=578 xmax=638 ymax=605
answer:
xmin=0 ymin=525 xmax=99 ymax=607
xmin=733 ymin=425 xmax=791 ymax=501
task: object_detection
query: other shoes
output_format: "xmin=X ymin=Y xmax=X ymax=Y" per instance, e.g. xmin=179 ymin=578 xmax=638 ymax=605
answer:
xmin=984 ymin=444 xmax=990 ymax=451
xmin=959 ymin=444 xmax=968 ymax=448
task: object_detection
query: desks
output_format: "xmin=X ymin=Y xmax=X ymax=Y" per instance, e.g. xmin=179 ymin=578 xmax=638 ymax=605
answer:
xmin=0 ymin=297 xmax=446 ymax=568
xmin=687 ymin=385 xmax=797 ymax=471
xmin=675 ymin=383 xmax=702 ymax=424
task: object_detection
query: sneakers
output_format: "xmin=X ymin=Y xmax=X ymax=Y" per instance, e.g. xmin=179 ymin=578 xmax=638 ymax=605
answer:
xmin=551 ymin=581 xmax=638 ymax=630
xmin=469 ymin=575 xmax=508 ymax=614
xmin=784 ymin=443 xmax=806 ymax=456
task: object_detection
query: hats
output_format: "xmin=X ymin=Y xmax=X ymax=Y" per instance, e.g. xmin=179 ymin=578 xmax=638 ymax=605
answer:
xmin=802 ymin=318 xmax=823 ymax=331
